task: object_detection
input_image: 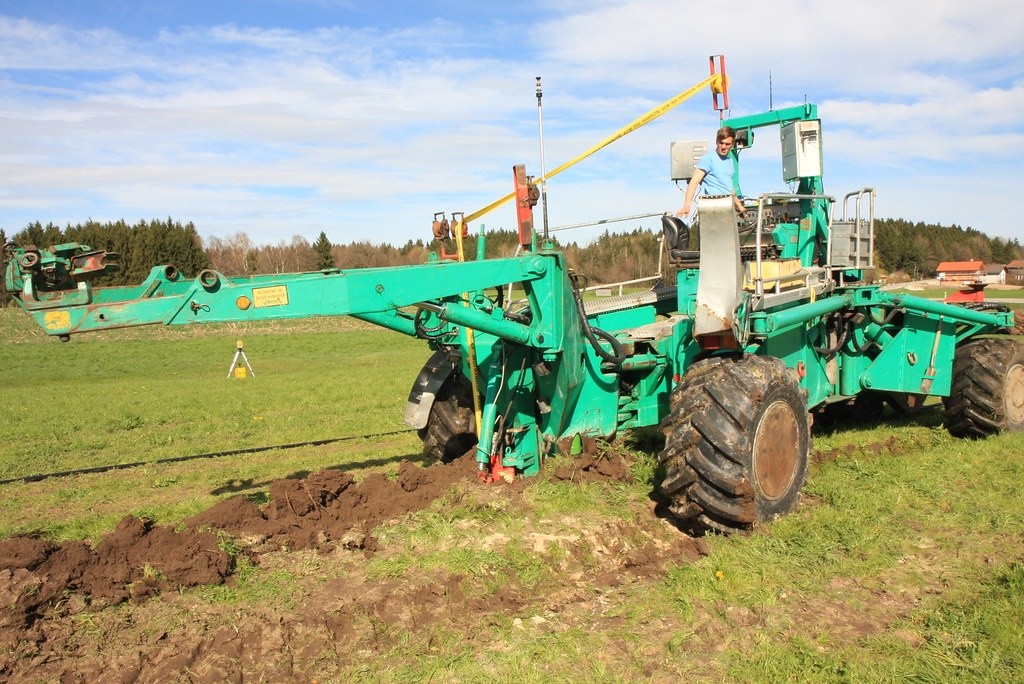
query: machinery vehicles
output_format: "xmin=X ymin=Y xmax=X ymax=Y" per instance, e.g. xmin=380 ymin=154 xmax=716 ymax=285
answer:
xmin=14 ymin=55 xmax=1024 ymax=541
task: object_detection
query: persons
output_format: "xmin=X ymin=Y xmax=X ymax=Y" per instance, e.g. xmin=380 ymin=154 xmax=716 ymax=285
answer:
xmin=673 ymin=126 xmax=753 ymax=224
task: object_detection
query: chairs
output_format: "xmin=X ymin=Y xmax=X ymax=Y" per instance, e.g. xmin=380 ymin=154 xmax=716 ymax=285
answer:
xmin=660 ymin=215 xmax=699 ymax=260
